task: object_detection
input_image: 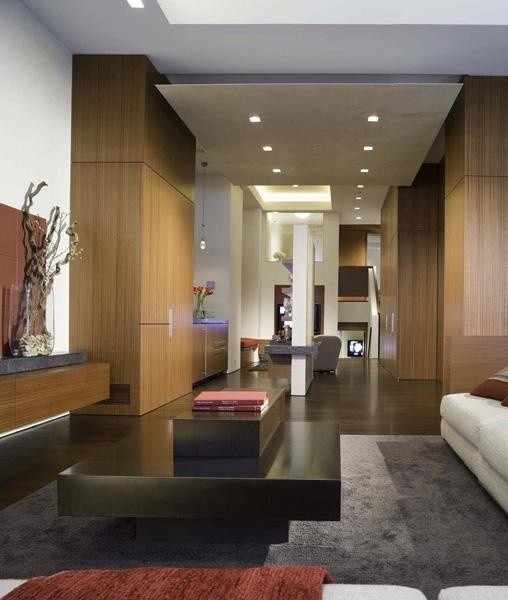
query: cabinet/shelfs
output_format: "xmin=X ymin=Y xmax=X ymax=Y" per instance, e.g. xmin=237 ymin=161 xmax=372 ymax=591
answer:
xmin=378 ymin=182 xmax=443 ymax=381
xmin=274 ymin=295 xmax=293 ymax=344
xmin=190 ymin=318 xmax=229 ymax=387
xmin=69 ymin=53 xmax=192 ymax=418
xmin=436 ymin=70 xmax=507 ymax=382
xmin=239 ymin=336 xmax=259 ymax=369
xmin=0 ymin=357 xmax=115 ymax=439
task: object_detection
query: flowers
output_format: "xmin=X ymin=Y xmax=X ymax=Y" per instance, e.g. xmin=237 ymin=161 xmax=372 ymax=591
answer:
xmin=272 ymin=251 xmax=287 ymax=258
xmin=190 ymin=283 xmax=214 ymax=311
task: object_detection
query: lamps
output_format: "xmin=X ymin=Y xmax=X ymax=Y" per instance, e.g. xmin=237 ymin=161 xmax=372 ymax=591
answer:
xmin=199 ymin=161 xmax=209 ymax=250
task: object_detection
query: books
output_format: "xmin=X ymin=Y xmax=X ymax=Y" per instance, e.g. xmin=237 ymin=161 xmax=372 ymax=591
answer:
xmin=193 ymin=400 xmax=268 ymax=411
xmin=193 ymin=391 xmax=268 ymax=405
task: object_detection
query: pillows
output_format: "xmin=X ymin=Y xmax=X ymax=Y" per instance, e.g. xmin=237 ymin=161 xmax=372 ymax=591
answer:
xmin=502 ymin=386 xmax=508 ymax=410
xmin=469 ymin=362 xmax=508 ymax=401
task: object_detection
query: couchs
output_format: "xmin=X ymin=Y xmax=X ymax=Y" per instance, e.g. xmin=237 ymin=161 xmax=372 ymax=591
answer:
xmin=437 ymin=333 xmax=508 ymax=516
xmin=439 ymin=576 xmax=507 ymax=600
xmin=0 ymin=564 xmax=431 ymax=600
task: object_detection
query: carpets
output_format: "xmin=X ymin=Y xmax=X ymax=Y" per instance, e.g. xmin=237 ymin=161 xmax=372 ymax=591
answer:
xmin=1 ymin=428 xmax=508 ymax=600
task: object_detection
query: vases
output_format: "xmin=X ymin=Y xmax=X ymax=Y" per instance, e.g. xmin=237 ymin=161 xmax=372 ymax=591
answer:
xmin=192 ymin=307 xmax=205 ymax=320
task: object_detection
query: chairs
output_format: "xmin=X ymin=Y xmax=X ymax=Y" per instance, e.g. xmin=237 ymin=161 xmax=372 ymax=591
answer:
xmin=312 ymin=334 xmax=343 ymax=376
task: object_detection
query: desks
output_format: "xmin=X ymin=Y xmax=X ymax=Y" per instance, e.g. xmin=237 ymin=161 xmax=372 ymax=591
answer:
xmin=51 ymin=388 xmax=344 ymax=542
